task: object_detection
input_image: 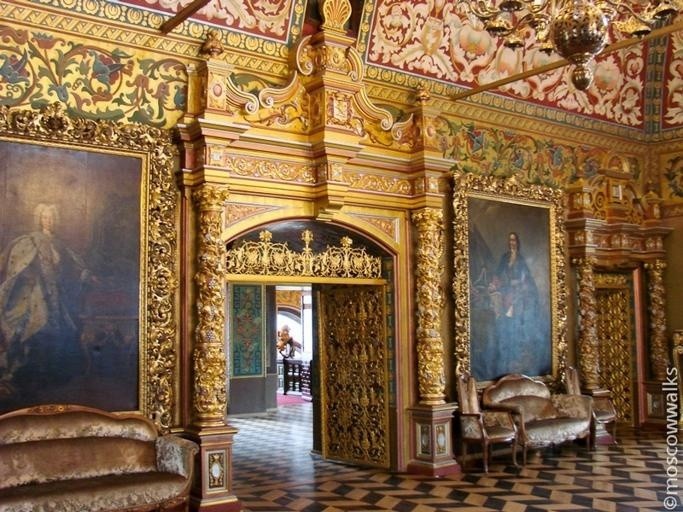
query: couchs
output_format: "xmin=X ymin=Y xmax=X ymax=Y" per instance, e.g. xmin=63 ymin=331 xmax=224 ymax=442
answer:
xmin=0 ymin=405 xmax=200 ymax=512
xmin=481 ymin=372 xmax=593 ymax=463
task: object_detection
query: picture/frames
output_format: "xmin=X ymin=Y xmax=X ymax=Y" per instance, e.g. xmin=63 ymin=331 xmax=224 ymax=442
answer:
xmin=0 ymin=105 xmax=174 ymax=434
xmin=451 ymin=171 xmax=569 ymax=397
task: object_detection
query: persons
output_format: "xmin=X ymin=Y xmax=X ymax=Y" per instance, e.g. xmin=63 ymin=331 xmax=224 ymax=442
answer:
xmin=1 ymin=200 xmax=98 ymax=390
xmin=490 ymin=229 xmax=538 ymax=299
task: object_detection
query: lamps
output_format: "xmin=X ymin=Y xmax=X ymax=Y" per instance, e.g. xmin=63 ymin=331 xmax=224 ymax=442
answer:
xmin=548 ymin=1 xmax=608 ymax=92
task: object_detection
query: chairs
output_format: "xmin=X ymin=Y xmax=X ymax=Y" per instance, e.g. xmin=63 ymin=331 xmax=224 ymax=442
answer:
xmin=563 ymin=367 xmax=620 ymax=447
xmin=454 ymin=377 xmax=517 ymax=471
